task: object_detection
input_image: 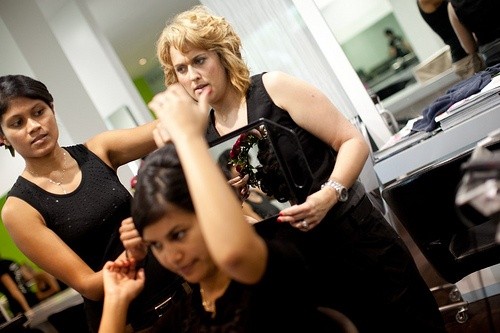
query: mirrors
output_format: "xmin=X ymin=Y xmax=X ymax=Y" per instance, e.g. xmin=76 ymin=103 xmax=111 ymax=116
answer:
xmin=293 ymin=0 xmax=500 ymax=149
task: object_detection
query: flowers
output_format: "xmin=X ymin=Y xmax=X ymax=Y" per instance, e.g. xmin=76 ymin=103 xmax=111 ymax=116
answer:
xmin=225 ymin=131 xmax=261 ymax=192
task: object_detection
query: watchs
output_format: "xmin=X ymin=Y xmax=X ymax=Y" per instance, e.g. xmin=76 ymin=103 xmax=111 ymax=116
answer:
xmin=325 ymin=179 xmax=349 ymax=201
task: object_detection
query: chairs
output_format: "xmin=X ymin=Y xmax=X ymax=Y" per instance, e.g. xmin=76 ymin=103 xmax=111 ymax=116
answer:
xmin=382 ymin=143 xmax=500 ymax=283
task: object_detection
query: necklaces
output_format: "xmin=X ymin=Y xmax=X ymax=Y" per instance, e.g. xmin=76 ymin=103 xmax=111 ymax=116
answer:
xmin=26 ymin=149 xmax=68 ymax=194
xmin=199 ymin=287 xmax=214 ymax=310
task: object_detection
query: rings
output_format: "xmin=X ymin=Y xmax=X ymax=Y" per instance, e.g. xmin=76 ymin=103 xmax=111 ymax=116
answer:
xmin=303 ymin=221 xmax=309 ymax=228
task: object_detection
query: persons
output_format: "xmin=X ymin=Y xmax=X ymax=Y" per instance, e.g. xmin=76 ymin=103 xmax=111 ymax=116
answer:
xmin=158 ymin=7 xmax=450 ymax=333
xmin=21 ymin=263 xmax=68 ymax=299
xmin=97 ymin=82 xmax=324 ymax=333
xmin=0 ymin=257 xmax=39 ymax=318
xmin=417 ymin=0 xmax=500 ymax=63
xmin=216 ymin=149 xmax=281 ymax=220
xmin=0 ymin=74 xmax=159 ymax=333
xmin=384 ymin=29 xmax=410 ymax=56
